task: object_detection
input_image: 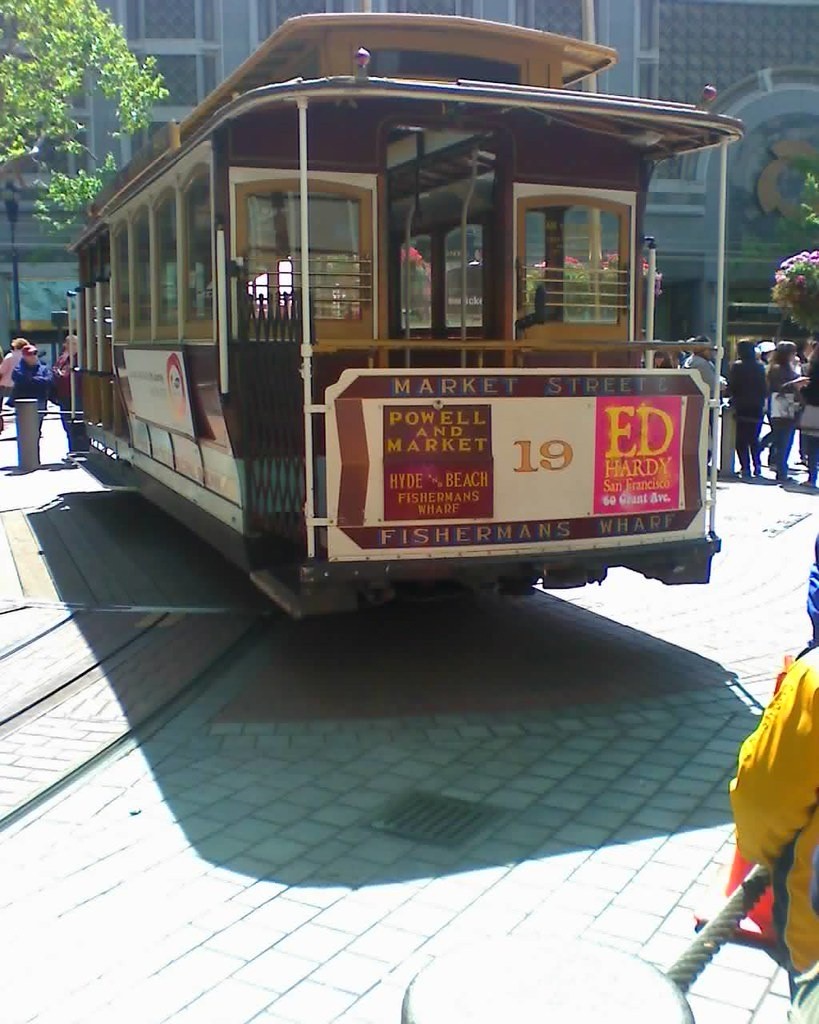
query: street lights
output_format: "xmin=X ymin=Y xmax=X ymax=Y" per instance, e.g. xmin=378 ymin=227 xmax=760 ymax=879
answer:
xmin=4 ymin=180 xmax=21 ymax=336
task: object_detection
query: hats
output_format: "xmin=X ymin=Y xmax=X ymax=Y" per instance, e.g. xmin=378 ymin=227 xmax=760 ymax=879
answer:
xmin=758 ymin=342 xmax=775 ymax=353
xmin=22 ymin=346 xmax=38 ymax=354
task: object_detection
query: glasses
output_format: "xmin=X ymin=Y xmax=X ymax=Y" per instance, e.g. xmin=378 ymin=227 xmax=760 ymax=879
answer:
xmin=28 ymin=352 xmax=37 ymax=355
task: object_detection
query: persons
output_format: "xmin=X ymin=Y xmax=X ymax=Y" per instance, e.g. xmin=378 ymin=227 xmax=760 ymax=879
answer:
xmin=0 ymin=335 xmax=78 ymax=461
xmin=642 ymin=335 xmax=819 ymax=488
xmin=730 ymin=533 xmax=819 ymax=1024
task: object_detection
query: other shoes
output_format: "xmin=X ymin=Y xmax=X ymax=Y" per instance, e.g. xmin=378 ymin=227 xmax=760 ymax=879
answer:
xmin=718 ymin=467 xmax=819 ymax=492
xmin=794 ymin=462 xmax=806 ymax=465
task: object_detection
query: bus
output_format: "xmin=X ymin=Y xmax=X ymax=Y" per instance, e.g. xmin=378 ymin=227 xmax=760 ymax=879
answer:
xmin=68 ymin=13 xmax=746 ymax=624
